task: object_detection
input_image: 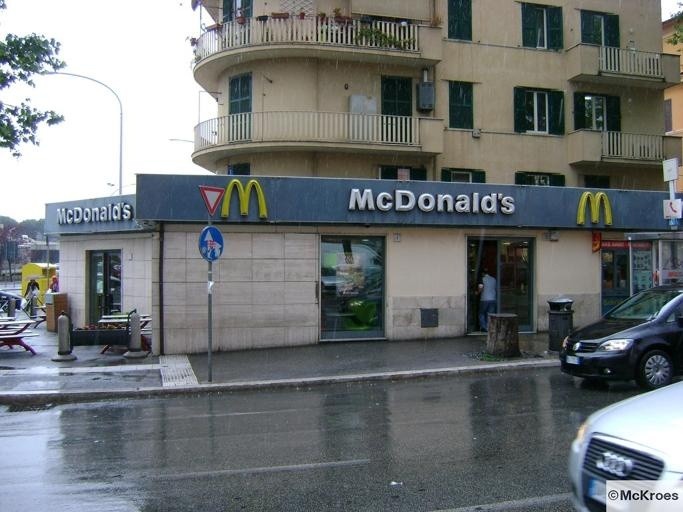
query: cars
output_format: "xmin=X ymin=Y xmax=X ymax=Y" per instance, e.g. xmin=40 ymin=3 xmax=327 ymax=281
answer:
xmin=562 ymin=368 xmax=683 ymax=510
xmin=557 ymin=282 xmax=683 ymax=390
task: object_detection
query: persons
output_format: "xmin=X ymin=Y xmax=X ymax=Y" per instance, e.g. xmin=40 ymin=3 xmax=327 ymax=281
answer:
xmin=23 ymin=279 xmax=40 ymax=311
xmin=49 ymin=275 xmax=59 ymax=293
xmin=25 ymin=281 xmax=38 ymax=313
xmin=473 ymin=266 xmax=496 ymax=332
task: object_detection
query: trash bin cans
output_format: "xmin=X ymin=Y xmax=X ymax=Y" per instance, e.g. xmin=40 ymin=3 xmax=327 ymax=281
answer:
xmin=547 ymin=298 xmax=574 ymax=356
xmin=45 ymin=292 xmax=67 ymax=333
xmin=21 ymin=262 xmax=59 ymax=307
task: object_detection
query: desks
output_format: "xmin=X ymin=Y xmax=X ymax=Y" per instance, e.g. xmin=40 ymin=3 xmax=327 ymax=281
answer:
xmin=96 ymin=314 xmax=151 ymax=355
xmin=0 ymin=316 xmax=35 ymax=351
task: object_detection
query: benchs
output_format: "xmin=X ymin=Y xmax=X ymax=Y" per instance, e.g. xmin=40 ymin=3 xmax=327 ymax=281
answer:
xmin=0 ymin=333 xmax=39 ymax=339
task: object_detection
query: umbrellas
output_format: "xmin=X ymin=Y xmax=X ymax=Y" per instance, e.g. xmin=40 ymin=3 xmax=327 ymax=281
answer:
xmin=24 ymin=274 xmax=42 ymax=281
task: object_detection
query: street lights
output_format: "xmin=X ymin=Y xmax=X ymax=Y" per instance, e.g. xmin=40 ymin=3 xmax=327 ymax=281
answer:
xmin=37 ymin=69 xmax=123 ymax=198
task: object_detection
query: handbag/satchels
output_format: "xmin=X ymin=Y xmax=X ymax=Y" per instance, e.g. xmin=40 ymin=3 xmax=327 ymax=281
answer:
xmin=32 ymin=287 xmax=40 ymax=298
xmin=20 ymin=298 xmax=31 ymax=311
